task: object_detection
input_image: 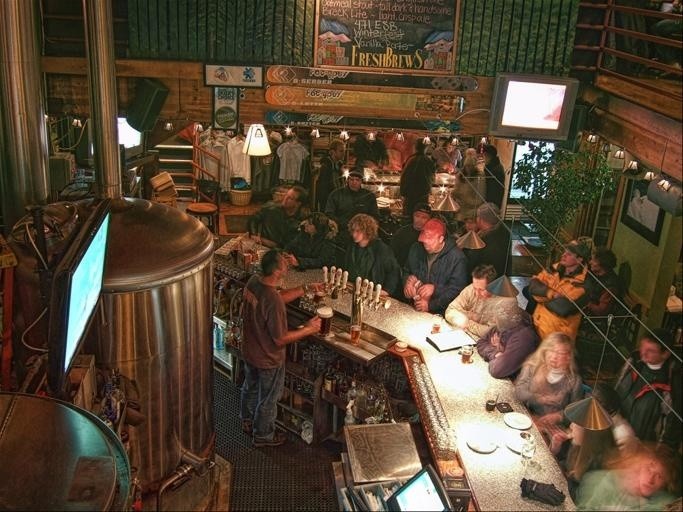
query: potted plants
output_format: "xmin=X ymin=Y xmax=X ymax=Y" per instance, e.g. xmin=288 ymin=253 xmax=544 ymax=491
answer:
xmin=268 ymin=178 xmax=297 ymax=202
xmin=228 ymin=179 xmax=251 ymax=206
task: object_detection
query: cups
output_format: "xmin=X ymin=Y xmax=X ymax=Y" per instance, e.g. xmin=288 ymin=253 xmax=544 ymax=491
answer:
xmin=521 ymin=442 xmax=535 ymax=473
xmin=432 ymin=314 xmax=443 ymax=333
xmin=485 ymin=388 xmax=499 ymax=412
xmin=350 ymin=325 xmax=361 ymax=346
xmin=462 ymin=345 xmax=473 ymax=364
xmin=316 ymin=307 xmax=333 ymax=337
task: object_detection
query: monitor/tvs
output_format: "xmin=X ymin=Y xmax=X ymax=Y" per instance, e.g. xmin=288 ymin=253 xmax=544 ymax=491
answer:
xmin=43 ymin=198 xmax=115 ymax=393
xmin=488 ymin=73 xmax=580 ymax=142
xmin=506 ymin=143 xmax=554 ymax=198
xmin=387 ymin=465 xmax=455 ymax=512
xmin=124 ymin=79 xmax=167 ymax=133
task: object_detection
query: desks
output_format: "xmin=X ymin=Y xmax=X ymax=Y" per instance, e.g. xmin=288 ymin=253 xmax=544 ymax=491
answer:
xmin=661 ymin=296 xmax=682 ymax=340
xmin=363 ymin=170 xmax=455 ymax=189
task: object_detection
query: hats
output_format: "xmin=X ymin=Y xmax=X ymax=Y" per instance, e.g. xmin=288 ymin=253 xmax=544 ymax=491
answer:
xmin=564 ymin=235 xmax=592 ymax=257
xmin=349 ymin=170 xmax=363 ymax=178
xmin=418 ymin=218 xmax=446 ymax=243
xmin=412 ymin=201 xmax=430 ymax=212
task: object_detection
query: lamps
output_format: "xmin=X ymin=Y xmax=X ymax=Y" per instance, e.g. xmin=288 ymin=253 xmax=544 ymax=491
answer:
xmin=434 ymin=148 xmax=460 ymax=212
xmin=309 ymin=128 xmax=319 ymax=138
xmin=563 ymin=314 xmax=614 ymax=431
xmin=450 ymin=137 xmax=459 ymax=146
xmin=194 ymin=123 xmax=204 ymax=131
xmin=284 ymin=127 xmax=293 ymax=135
xmin=455 ymin=178 xmax=486 ymax=249
xmin=255 ymin=128 xmax=262 ymax=138
xmin=480 ymin=137 xmax=487 ymax=144
xmin=70 ymin=116 xmax=82 ymax=127
xmin=483 ymin=216 xmax=519 ymax=298
xmin=656 ymin=180 xmax=669 ymax=191
xmin=585 ymin=134 xmax=596 ymax=143
xmin=421 ymin=136 xmax=431 ymax=145
xmin=339 ymin=131 xmax=349 ymax=141
xmin=394 ymin=132 xmax=404 ymax=141
xmin=366 ymin=132 xmax=375 ymax=141
xmin=164 ymin=122 xmax=173 ymax=131
xmin=643 ymin=171 xmax=654 ymax=181
xmin=600 ymin=142 xmax=610 ymax=152
xmin=626 ymin=160 xmax=637 ymax=170
xmin=612 ymin=148 xmax=624 ymax=159
xmin=241 ymin=124 xmax=272 ymax=156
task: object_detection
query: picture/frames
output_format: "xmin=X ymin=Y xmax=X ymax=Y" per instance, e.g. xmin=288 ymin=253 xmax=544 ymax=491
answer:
xmin=618 ymin=178 xmax=665 ymax=247
xmin=313 ymin=0 xmax=458 ymax=78
xmin=201 ymin=61 xmax=264 ymax=89
xmin=212 ymin=86 xmax=240 ymax=131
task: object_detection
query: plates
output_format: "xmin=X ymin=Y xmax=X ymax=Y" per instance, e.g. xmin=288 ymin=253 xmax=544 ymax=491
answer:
xmin=504 ymin=412 xmax=533 ymax=430
xmin=465 ymin=428 xmax=497 ymax=453
xmin=504 ymin=430 xmax=535 ymax=455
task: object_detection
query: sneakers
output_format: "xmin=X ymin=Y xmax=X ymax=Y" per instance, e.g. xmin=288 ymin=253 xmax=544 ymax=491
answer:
xmin=254 ymin=431 xmax=287 ymax=446
xmin=242 ymin=419 xmax=254 ymax=432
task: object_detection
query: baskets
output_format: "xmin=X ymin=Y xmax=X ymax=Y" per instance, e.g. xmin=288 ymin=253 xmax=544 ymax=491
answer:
xmin=271 ymin=191 xmax=287 ymax=201
xmin=230 ymin=189 xmax=252 ymax=206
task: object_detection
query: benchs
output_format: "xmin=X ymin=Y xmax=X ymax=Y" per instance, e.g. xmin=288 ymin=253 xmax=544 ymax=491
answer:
xmin=218 ymin=202 xmax=266 ymax=235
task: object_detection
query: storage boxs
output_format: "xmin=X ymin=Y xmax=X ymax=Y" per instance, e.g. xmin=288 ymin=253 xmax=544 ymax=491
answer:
xmin=150 ymin=198 xmax=178 ymax=209
xmin=149 ymin=171 xmax=177 ymax=199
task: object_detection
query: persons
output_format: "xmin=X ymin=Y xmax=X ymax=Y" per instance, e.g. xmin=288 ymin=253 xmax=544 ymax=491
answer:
xmin=528 ymin=239 xmax=589 ymax=344
xmin=462 ymin=201 xmax=513 ymax=287
xmin=315 ymin=139 xmax=345 ymax=213
xmin=512 ymin=330 xmax=582 ymax=416
xmin=400 ymin=138 xmax=434 ymax=216
xmin=238 ymin=248 xmax=329 ymax=447
xmin=389 ymin=203 xmax=434 ymax=268
xmin=584 ymin=246 xmax=620 ymax=315
xmin=405 ymin=273 xmax=447 ymax=300
xmin=280 ymin=211 xmax=339 ymax=272
xmin=245 ymin=185 xmax=311 ymax=248
xmin=452 ymin=156 xmax=487 ymax=212
xmin=482 ymin=144 xmax=506 ymax=210
xmin=453 ymin=211 xmax=477 ymax=239
xmin=613 ymin=328 xmax=674 ymax=446
xmin=444 ymin=263 xmax=518 ymax=341
xmin=325 ymin=167 xmax=380 ymax=230
xmin=431 ymin=140 xmax=462 ymax=174
xmin=536 ymin=380 xmax=640 ymax=451
xmin=573 ymin=441 xmax=682 ymax=512
xmin=550 ymin=422 xmax=614 ymax=497
xmin=477 ymin=296 xmax=540 ymax=379
xmin=341 ymin=213 xmax=404 ymax=298
xmin=401 ymin=219 xmax=469 ymax=313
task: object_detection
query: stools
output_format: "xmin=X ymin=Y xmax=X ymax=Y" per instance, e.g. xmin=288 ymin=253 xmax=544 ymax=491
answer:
xmin=185 ymin=203 xmax=217 ymax=236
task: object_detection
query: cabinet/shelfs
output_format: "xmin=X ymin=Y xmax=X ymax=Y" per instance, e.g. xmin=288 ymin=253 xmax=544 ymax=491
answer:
xmin=275 ymin=351 xmax=343 ymax=445
xmin=321 ymin=357 xmax=413 ymax=452
xmin=153 ymin=143 xmax=194 ymax=197
